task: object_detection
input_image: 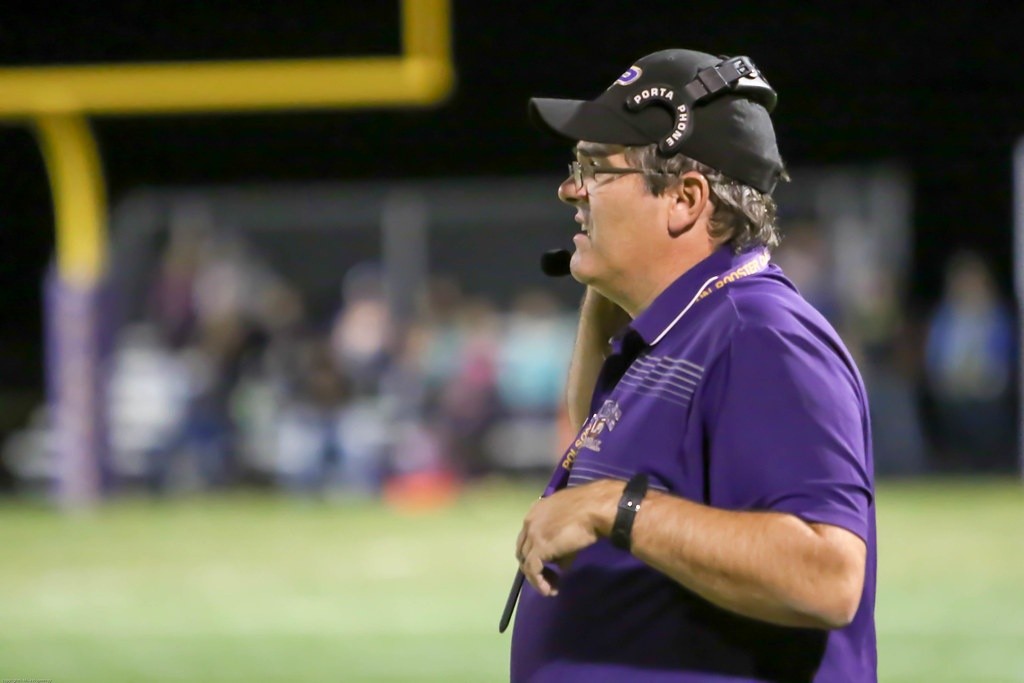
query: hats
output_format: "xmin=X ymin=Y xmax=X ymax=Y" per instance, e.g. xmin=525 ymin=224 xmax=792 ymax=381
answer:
xmin=530 ymin=48 xmax=784 ymax=193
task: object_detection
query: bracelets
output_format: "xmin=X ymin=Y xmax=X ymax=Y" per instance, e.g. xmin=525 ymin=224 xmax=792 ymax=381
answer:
xmin=612 ymin=477 xmax=648 ymax=551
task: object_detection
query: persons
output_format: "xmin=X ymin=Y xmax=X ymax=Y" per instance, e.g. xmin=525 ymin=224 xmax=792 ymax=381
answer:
xmin=102 ymin=202 xmax=1022 ymax=492
xmin=510 ymin=49 xmax=876 ymax=683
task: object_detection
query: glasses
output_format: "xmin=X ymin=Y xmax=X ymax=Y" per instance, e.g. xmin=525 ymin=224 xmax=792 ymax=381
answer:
xmin=567 ymin=161 xmax=680 ymax=190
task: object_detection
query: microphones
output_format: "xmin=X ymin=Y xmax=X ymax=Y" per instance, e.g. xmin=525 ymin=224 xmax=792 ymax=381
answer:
xmin=540 ymin=247 xmax=576 ymax=278
